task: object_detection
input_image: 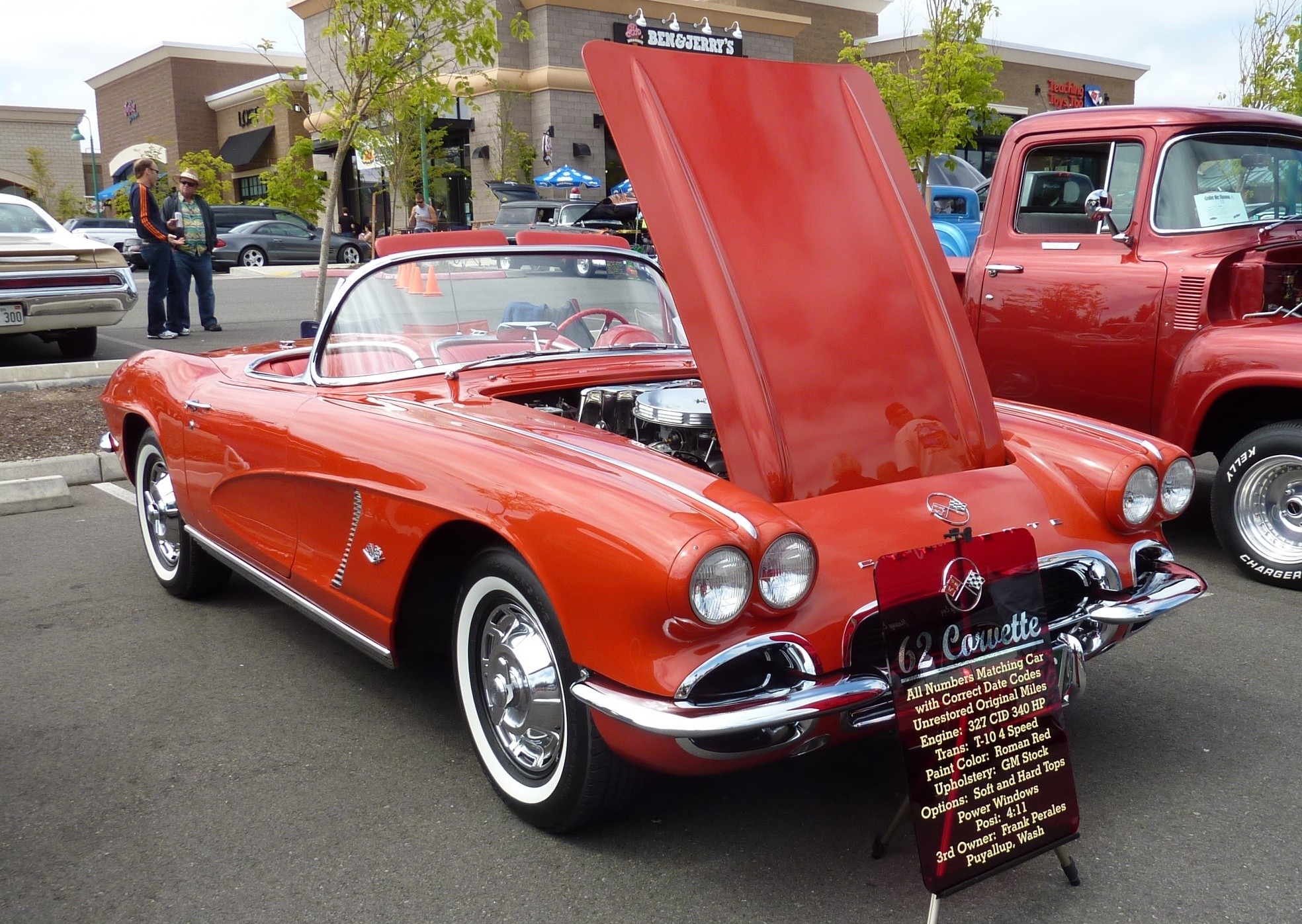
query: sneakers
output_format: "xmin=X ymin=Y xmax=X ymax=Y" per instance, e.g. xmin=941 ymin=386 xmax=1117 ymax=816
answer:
xmin=180 ymin=327 xmax=189 ymax=335
xmin=206 ymin=324 xmax=223 ymax=331
xmin=147 ymin=329 xmax=178 ymax=339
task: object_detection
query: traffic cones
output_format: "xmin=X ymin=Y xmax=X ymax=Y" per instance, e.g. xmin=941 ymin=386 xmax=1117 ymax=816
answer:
xmin=394 ymin=262 xmax=416 ymax=291
xmin=422 ymin=264 xmax=442 ymax=297
xmin=409 ymin=266 xmax=426 ymax=295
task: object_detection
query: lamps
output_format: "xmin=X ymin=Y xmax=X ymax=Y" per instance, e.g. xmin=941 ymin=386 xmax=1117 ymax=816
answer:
xmin=724 ymin=21 xmax=743 ymax=39
xmin=662 ymin=12 xmax=680 ymax=31
xmin=694 ymin=17 xmax=712 ymax=35
xmin=629 ymin=8 xmax=647 ymax=27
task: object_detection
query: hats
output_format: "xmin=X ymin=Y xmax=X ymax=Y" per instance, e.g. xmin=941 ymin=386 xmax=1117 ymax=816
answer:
xmin=172 ymin=169 xmax=204 ymax=189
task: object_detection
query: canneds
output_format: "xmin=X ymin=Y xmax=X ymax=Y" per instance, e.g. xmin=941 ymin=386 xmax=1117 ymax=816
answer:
xmin=174 ymin=212 xmax=183 ymax=228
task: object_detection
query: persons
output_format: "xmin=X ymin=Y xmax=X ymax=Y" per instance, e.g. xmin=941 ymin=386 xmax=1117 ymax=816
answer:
xmin=409 ymin=194 xmax=437 ymax=233
xmin=338 ymin=207 xmax=372 ymax=245
xmin=160 ymin=169 xmax=222 ymax=331
xmin=130 ymin=158 xmax=190 ymax=338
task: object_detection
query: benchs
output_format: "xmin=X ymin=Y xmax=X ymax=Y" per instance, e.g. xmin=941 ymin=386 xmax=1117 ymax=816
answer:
xmin=1014 ymin=213 xmax=1171 ymax=239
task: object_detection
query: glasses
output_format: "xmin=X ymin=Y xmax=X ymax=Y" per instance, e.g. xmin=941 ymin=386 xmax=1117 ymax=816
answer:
xmin=149 ymin=168 xmax=159 ymax=174
xmin=180 ymin=181 xmax=197 ymax=187
xmin=416 ymin=200 xmax=423 ymax=203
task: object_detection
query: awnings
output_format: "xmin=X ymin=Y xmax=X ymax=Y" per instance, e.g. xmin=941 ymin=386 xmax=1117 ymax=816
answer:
xmin=98 ymin=173 xmax=168 ymax=200
xmin=573 ymin=143 xmax=591 ymax=157
xmin=472 ymin=146 xmax=489 ymax=159
xmin=216 ymin=125 xmax=274 ymax=167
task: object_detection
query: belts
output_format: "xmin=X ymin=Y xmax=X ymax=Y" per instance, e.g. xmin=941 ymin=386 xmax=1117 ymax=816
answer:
xmin=141 ymin=238 xmax=160 ymax=243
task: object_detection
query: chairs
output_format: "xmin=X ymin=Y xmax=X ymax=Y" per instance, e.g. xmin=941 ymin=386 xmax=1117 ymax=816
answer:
xmin=432 ymin=336 xmax=578 ymax=366
xmin=245 ymin=341 xmax=423 ymax=378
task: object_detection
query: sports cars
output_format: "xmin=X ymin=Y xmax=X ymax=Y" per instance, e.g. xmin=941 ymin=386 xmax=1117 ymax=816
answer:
xmin=95 ymin=36 xmax=1206 ymax=843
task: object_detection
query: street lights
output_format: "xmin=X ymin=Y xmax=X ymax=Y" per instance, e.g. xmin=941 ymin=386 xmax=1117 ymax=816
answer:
xmin=70 ymin=114 xmax=100 ymax=218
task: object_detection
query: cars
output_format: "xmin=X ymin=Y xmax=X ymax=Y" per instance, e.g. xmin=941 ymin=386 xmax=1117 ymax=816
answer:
xmin=63 ymin=217 xmax=149 ymax=273
xmin=0 ymin=193 xmax=140 ymax=361
xmin=479 ymin=179 xmax=639 ymax=245
xmin=913 ymin=151 xmax=1097 ymax=279
xmin=211 ymin=219 xmax=372 ymax=272
xmin=937 ymin=99 xmax=1302 ymax=588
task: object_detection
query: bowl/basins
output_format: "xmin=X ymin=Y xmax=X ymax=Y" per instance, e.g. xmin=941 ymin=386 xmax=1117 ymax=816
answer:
xmin=415 ymin=215 xmax=420 ymax=219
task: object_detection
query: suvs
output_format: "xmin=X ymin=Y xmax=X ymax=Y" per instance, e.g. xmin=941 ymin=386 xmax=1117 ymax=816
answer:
xmin=209 ymin=202 xmax=316 ymax=235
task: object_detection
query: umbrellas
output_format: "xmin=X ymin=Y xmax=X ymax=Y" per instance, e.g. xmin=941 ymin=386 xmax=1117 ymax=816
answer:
xmin=611 ymin=178 xmax=633 ymax=195
xmin=534 ymin=164 xmax=601 ymax=199
